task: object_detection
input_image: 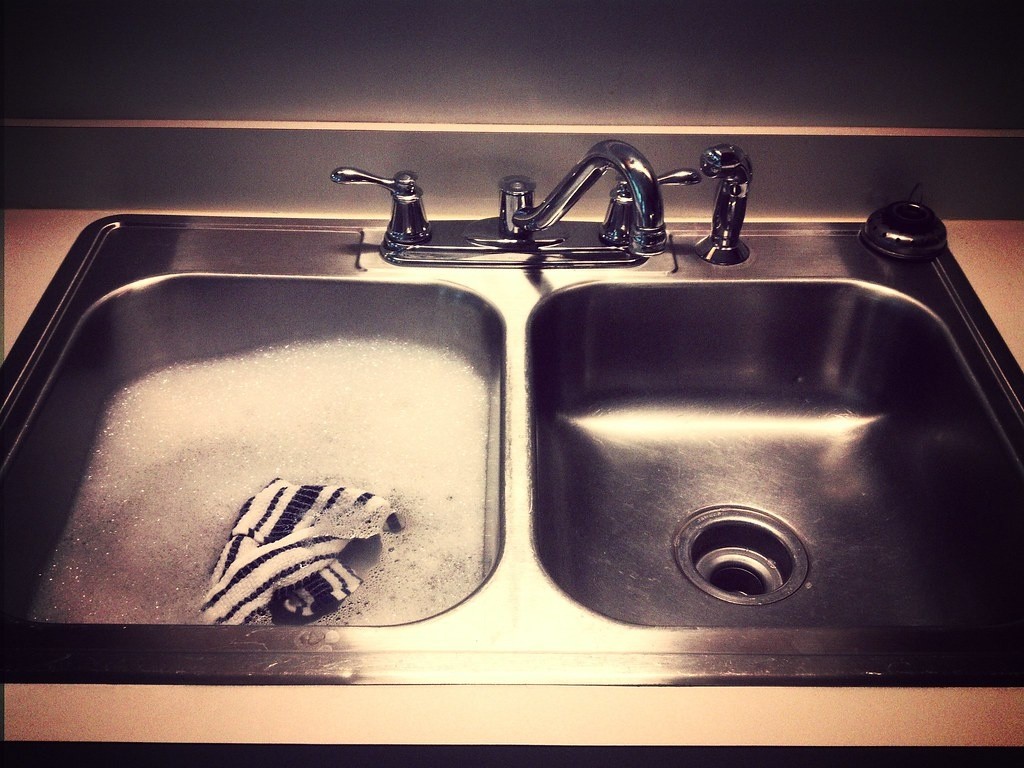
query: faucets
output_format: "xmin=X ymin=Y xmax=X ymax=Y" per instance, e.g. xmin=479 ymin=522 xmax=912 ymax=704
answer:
xmin=497 ymin=139 xmax=669 ymax=258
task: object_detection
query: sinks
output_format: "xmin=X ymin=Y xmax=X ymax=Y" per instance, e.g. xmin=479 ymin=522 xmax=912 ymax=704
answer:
xmin=517 ymin=221 xmax=1024 ymax=689
xmin=0 ymin=213 xmax=517 ymax=686
